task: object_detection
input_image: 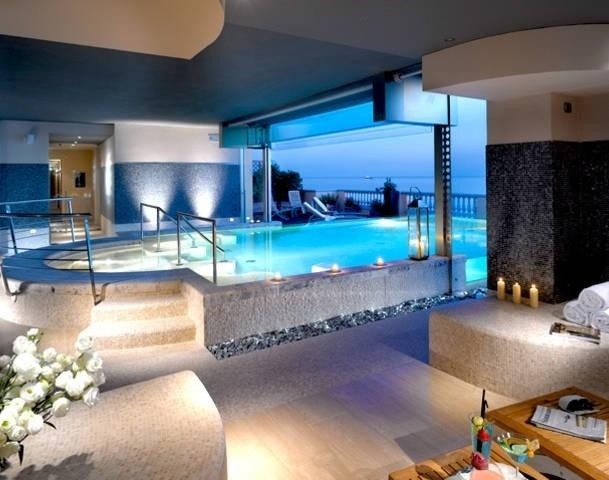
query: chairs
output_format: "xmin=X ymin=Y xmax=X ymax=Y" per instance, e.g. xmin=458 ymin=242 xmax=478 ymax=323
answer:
xmin=489 ymin=386 xmax=609 ymax=480
xmin=271 ymin=189 xmax=370 ymax=222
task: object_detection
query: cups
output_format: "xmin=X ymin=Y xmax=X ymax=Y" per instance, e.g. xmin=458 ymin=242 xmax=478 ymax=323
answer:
xmin=468 ymin=412 xmax=496 ymax=463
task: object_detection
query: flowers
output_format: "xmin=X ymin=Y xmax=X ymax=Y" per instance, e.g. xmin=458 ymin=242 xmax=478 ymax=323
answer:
xmin=0 ymin=325 xmax=105 ymax=476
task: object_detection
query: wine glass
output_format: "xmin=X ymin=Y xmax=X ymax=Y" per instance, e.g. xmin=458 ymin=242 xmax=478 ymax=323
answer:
xmin=495 ymin=432 xmax=534 ymax=480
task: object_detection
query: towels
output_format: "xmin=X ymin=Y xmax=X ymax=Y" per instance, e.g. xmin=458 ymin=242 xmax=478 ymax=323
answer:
xmin=564 ymin=282 xmax=609 ymax=334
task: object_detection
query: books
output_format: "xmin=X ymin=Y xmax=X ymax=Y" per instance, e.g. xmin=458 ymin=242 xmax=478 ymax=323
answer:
xmin=552 ymin=322 xmax=601 ymax=345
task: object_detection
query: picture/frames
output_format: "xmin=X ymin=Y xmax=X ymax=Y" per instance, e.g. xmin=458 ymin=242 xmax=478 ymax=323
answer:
xmin=72 ymin=170 xmax=88 ymax=190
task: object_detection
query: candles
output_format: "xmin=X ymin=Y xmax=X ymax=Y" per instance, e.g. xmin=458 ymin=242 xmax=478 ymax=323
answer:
xmin=377 ymin=258 xmax=385 ymax=266
xmin=497 ymin=275 xmax=506 ymax=300
xmin=331 ymin=263 xmax=339 ymax=273
xmin=275 ymin=272 xmax=282 ymax=281
xmin=512 ymin=281 xmax=521 ymax=304
xmin=416 ymin=235 xmax=424 ymax=258
xmin=529 ymin=284 xmax=538 ymax=309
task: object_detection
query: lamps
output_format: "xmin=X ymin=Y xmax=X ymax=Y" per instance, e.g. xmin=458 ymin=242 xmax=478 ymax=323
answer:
xmin=25 ymin=133 xmax=36 ymax=145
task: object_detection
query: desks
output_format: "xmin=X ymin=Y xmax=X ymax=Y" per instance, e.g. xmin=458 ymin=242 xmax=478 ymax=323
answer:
xmin=388 ymin=440 xmax=552 ymax=480
